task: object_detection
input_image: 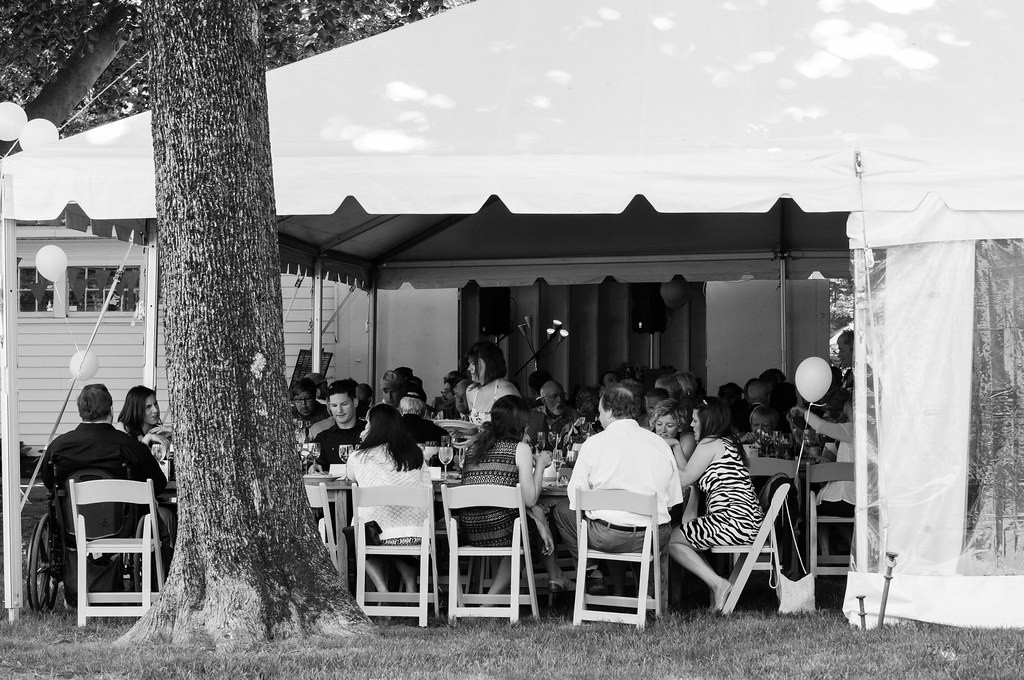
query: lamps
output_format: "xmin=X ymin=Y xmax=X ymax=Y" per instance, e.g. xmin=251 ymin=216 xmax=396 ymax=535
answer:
xmin=515 ymin=314 xmax=569 ymax=376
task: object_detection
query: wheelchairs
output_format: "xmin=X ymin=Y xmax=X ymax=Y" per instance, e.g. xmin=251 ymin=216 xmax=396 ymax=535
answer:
xmin=26 ymin=457 xmax=174 ymax=620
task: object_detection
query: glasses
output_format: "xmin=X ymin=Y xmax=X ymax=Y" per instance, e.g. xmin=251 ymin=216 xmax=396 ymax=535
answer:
xmin=545 ymin=392 xmax=567 ymax=399
xmin=295 ymin=396 xmax=314 ymax=401
xmin=453 ymin=393 xmax=466 ymax=400
xmin=440 ymin=389 xmax=451 ymax=394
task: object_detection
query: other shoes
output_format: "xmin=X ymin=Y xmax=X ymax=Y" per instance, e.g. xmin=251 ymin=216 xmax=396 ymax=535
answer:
xmin=586 ymin=569 xmax=609 ymax=595
xmin=669 ymin=577 xmax=683 ymax=605
xmin=713 ymin=577 xmax=734 ymax=615
xmin=548 ymin=572 xmax=576 ymax=593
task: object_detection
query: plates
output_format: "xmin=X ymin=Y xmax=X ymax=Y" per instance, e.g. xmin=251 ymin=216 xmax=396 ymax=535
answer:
xmin=432 ymin=419 xmax=481 ymax=436
xmin=302 ymin=476 xmax=338 ymax=481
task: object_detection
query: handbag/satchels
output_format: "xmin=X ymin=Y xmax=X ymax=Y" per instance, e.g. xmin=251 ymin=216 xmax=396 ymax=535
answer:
xmin=64 ymin=549 xmax=125 ymax=608
xmin=777 ymin=572 xmax=815 ymax=614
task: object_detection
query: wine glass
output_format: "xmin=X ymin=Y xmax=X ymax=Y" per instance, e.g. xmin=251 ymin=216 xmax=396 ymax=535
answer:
xmin=528 ymin=431 xmax=578 ymax=487
xmin=416 ymin=435 xmax=468 ymax=481
xmin=761 ymin=428 xmax=820 ymax=460
xmin=294 ymin=419 xmax=361 ymax=474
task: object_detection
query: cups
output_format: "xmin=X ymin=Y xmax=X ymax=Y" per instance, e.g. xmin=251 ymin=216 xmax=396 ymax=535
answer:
xmin=152 ymin=443 xmax=165 ymax=463
xmin=431 ymin=411 xmax=441 ymax=420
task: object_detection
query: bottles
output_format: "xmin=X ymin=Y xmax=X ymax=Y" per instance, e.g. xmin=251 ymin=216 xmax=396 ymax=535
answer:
xmin=822 ymin=442 xmax=837 ymax=463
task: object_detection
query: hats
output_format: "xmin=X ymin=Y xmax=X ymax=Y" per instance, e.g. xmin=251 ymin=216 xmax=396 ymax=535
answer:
xmin=380 ymin=369 xmax=403 ymax=389
xmin=304 ymin=372 xmax=333 ymax=385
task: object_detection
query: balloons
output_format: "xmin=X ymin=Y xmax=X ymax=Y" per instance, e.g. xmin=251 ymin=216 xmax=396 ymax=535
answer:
xmin=795 ymin=357 xmax=833 ymax=402
xmin=35 ymin=245 xmax=68 ymax=281
xmin=70 ymin=350 xmax=98 ymax=381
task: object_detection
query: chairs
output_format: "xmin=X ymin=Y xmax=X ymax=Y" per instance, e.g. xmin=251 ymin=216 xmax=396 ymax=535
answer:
xmin=69 ymin=458 xmax=880 ymax=634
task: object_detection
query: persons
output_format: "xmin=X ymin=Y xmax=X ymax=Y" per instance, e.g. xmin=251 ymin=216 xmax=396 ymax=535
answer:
xmin=42 ymin=329 xmax=856 ymax=614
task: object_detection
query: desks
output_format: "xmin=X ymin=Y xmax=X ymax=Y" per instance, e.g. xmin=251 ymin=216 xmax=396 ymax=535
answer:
xmin=294 ymin=468 xmax=797 ymax=625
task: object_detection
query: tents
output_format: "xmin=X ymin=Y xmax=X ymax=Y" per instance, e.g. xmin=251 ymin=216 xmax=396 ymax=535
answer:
xmin=0 ymin=0 xmax=1024 ymax=624
